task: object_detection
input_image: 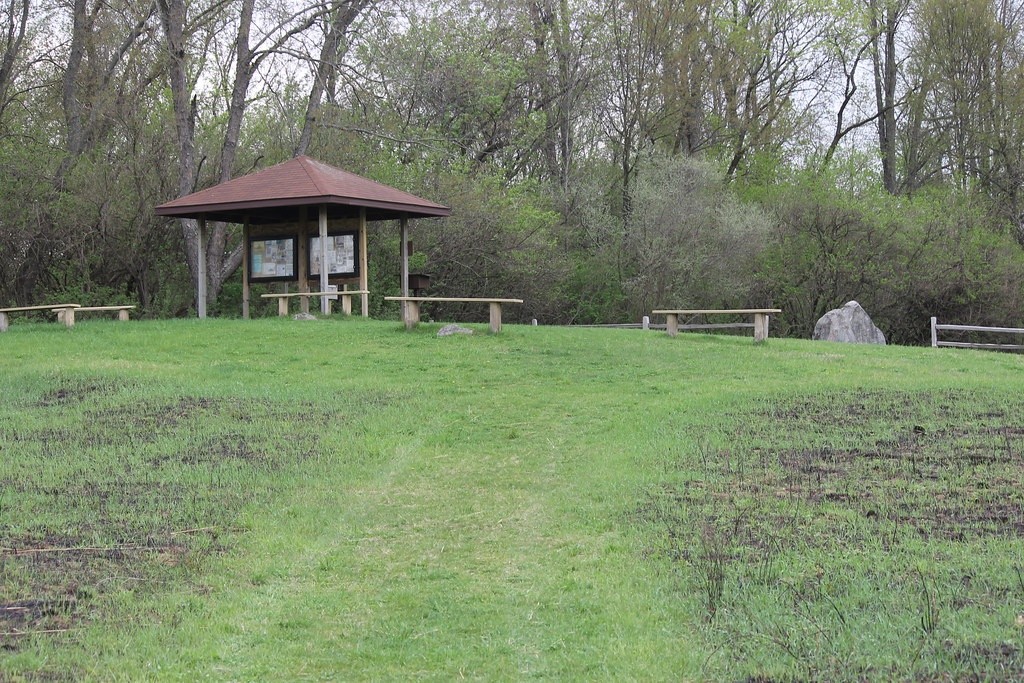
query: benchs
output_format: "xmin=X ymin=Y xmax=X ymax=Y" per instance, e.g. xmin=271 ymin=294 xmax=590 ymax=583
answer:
xmin=384 ymin=296 xmax=523 ymax=333
xmin=0 ymin=304 xmax=81 ymax=331
xmin=261 ymin=291 xmax=370 ymax=315
xmin=652 ymin=309 xmax=782 ymax=340
xmin=52 ymin=306 xmax=136 ymax=323
